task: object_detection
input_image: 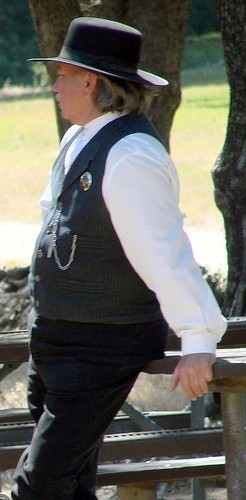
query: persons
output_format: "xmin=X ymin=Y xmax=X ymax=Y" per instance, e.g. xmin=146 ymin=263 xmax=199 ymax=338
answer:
xmin=12 ymin=15 xmax=228 ymax=500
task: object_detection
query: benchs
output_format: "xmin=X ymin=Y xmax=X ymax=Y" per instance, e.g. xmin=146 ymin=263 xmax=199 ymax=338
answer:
xmin=0 ymin=409 xmax=228 ymax=500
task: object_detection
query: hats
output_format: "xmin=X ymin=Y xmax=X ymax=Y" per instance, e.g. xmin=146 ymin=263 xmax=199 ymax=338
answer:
xmin=27 ymin=17 xmax=170 ymax=87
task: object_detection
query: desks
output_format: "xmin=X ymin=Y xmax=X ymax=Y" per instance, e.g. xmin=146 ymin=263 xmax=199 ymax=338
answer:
xmin=142 ymin=345 xmax=246 ymax=500
xmin=0 ymin=315 xmax=246 ymax=500
